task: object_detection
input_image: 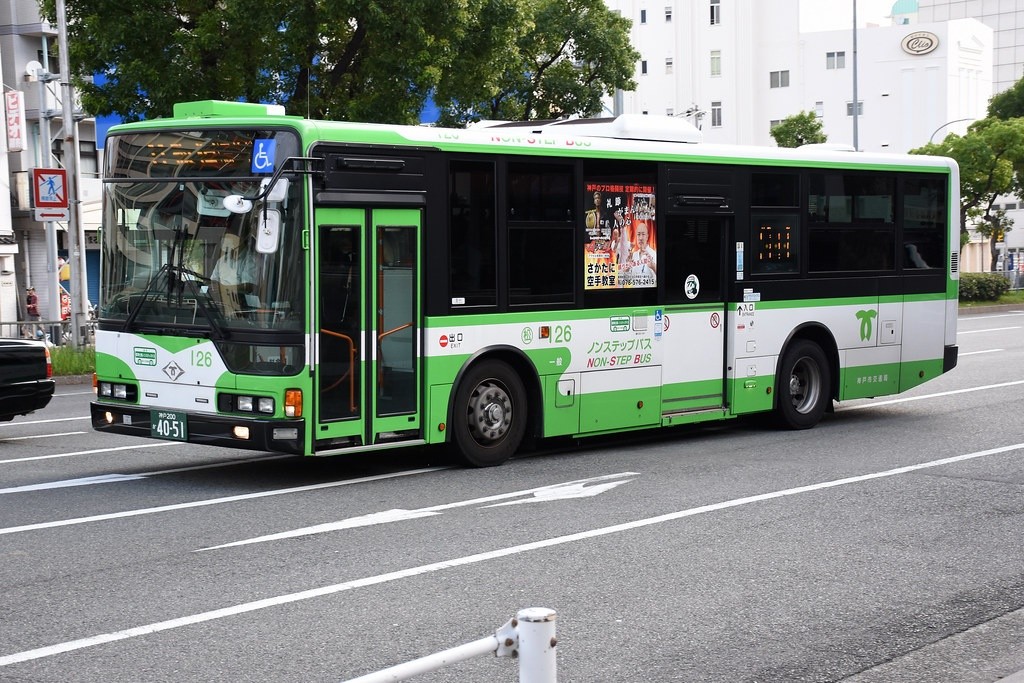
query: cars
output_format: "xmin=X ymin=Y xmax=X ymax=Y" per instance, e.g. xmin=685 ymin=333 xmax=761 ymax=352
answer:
xmin=0 ymin=337 xmax=55 ymax=421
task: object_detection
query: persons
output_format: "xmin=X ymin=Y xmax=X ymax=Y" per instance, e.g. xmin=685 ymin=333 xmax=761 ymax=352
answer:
xmin=585 ymin=191 xmax=658 ymax=288
xmin=25 ymin=286 xmax=46 ymax=339
xmin=203 ymin=230 xmax=260 ymax=299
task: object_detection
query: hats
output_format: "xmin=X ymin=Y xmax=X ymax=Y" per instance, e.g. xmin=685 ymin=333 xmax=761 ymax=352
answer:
xmin=26 ymin=286 xmax=35 ymax=291
xmin=221 ymin=234 xmax=240 ymax=251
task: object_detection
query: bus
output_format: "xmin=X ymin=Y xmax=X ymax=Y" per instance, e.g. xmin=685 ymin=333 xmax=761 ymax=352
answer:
xmin=90 ymin=98 xmax=961 ymax=469
